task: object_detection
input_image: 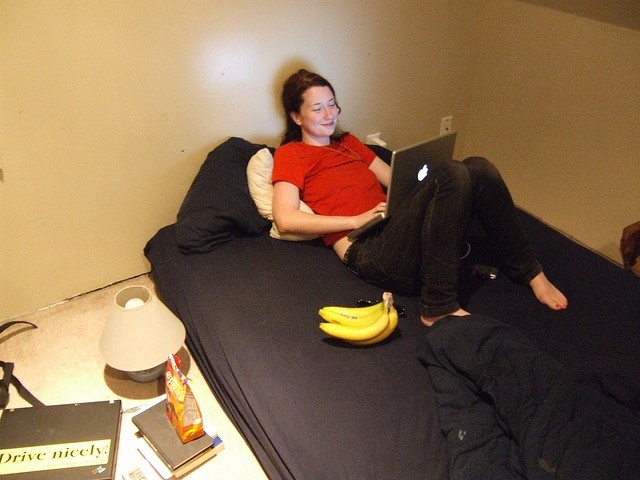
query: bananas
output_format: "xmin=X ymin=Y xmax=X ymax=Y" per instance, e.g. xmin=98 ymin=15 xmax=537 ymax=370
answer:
xmin=318 ymin=292 xmax=399 ymax=346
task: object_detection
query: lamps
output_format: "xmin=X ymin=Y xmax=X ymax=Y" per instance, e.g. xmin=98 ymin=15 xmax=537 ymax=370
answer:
xmin=103 ymin=287 xmax=187 ymax=382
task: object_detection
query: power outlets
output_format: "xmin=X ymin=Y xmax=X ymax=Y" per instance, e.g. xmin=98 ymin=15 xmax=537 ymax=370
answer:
xmin=365 ymin=132 xmax=381 ymax=150
xmin=441 ymin=115 xmax=454 ymax=137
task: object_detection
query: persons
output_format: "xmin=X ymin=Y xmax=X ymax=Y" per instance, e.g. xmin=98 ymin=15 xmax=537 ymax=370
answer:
xmin=271 ymin=68 xmax=568 ymax=327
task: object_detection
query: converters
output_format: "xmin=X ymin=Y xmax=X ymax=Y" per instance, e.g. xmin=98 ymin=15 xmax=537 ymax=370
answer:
xmin=371 ymin=136 xmax=387 ymax=149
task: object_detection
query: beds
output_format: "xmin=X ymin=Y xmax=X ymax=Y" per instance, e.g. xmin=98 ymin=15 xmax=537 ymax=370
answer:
xmin=149 ymin=145 xmax=636 ymax=480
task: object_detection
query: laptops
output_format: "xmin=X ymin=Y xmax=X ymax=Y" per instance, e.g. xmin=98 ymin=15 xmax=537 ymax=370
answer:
xmin=346 ymin=131 xmax=458 ymax=241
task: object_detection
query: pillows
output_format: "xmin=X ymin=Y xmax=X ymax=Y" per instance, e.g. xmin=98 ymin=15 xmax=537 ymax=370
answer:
xmin=247 ymin=146 xmax=317 ymax=243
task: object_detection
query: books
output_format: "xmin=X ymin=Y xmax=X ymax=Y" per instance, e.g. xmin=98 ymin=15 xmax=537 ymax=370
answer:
xmin=1 ymin=398 xmax=123 ymax=479
xmin=131 ymin=398 xmax=226 ymax=480
